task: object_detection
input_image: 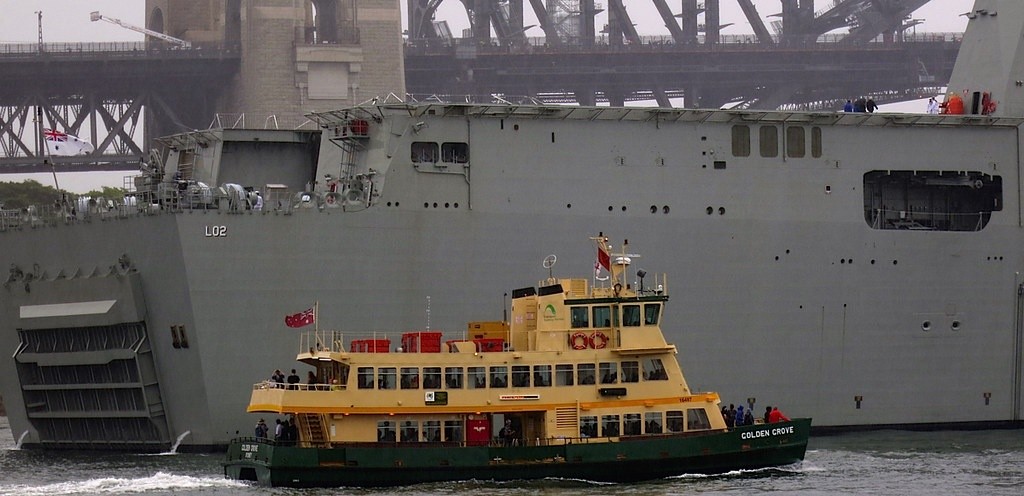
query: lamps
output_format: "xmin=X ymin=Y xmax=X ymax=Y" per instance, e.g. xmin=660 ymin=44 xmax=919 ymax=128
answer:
xmin=412 ymin=121 xmax=429 ymax=135
xmin=637 ymin=269 xmax=646 ymax=279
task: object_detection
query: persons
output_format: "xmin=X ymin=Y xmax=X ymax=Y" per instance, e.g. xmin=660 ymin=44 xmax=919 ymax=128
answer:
xmin=272 ymin=370 xmax=285 ymax=388
xmin=475 ymin=373 xmax=543 ymax=387
xmin=400 ymin=375 xmax=456 ymax=388
xmin=288 ymin=369 xmax=300 ymax=390
xmin=307 ymin=371 xmax=316 ymax=390
xmin=583 ymin=372 xmax=622 ymax=384
xmin=927 ymin=96 xmax=938 ymax=114
xmin=649 ymin=368 xmax=666 ymax=380
xmin=275 ymin=418 xmax=297 ymax=447
xmin=379 ymin=430 xmax=440 ymax=442
xmin=764 ymin=406 xmax=789 ymax=423
xmin=255 ymin=420 xmax=269 ymax=443
xmin=368 ymin=379 xmax=382 ymax=387
xmin=582 ymin=419 xmax=701 ymax=437
xmin=499 ymin=420 xmax=513 ymax=442
xmin=721 ymin=404 xmax=754 ymax=427
xmin=844 ymin=97 xmax=878 ymax=113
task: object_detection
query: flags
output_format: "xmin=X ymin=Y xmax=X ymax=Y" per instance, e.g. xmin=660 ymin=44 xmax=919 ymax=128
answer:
xmin=285 ymin=308 xmax=315 ymax=328
xmin=43 ymin=126 xmax=68 ymax=142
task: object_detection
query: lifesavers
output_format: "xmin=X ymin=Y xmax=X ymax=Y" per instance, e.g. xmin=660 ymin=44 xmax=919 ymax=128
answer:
xmin=589 ymin=332 xmax=606 ymax=349
xmin=571 ymin=332 xmax=588 ymax=350
xmin=988 ymin=101 xmax=996 ymax=112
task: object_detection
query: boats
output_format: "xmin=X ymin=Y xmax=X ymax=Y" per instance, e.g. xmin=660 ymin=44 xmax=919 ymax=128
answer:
xmin=1 ymin=0 xmax=1024 ymax=451
xmin=221 ymin=237 xmax=813 ymax=488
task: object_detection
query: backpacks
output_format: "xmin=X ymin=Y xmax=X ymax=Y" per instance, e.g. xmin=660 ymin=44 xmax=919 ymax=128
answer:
xmin=255 ymin=425 xmax=264 ymax=437
